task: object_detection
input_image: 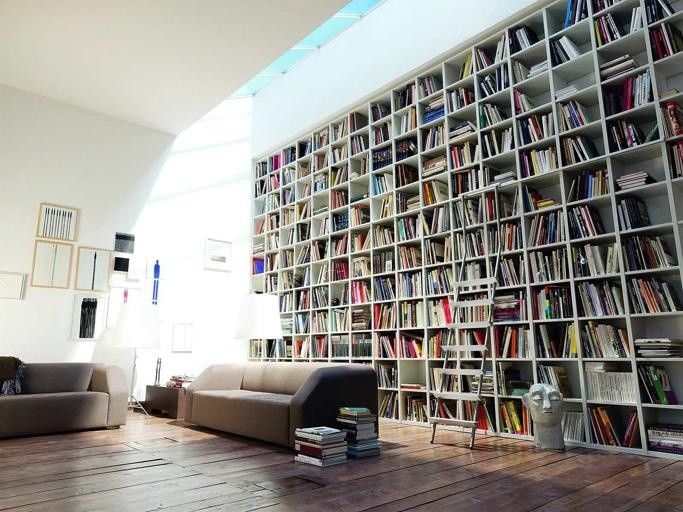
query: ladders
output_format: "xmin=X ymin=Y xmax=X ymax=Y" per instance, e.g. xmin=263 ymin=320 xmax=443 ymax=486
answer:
xmin=428 ymin=182 xmax=503 ymax=450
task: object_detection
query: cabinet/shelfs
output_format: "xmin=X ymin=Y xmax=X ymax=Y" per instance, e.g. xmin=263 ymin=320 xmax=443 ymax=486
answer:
xmin=249 ymin=0 xmax=681 ymax=459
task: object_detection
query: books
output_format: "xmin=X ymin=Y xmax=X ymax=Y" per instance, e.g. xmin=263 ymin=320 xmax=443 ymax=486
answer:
xmin=249 ymin=0 xmax=683 ymax=467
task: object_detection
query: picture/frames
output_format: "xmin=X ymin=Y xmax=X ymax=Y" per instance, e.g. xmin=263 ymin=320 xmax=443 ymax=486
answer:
xmin=202 ymin=236 xmax=233 ymax=273
xmin=28 ymin=201 xmax=111 ymax=344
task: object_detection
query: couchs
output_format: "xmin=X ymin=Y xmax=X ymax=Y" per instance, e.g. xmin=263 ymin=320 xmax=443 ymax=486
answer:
xmin=182 ymin=359 xmax=377 ymax=451
xmin=0 ymin=358 xmax=129 ymax=440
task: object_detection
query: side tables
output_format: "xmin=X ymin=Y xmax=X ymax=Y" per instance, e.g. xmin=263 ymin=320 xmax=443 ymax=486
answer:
xmin=143 ymin=383 xmax=186 ymax=418
